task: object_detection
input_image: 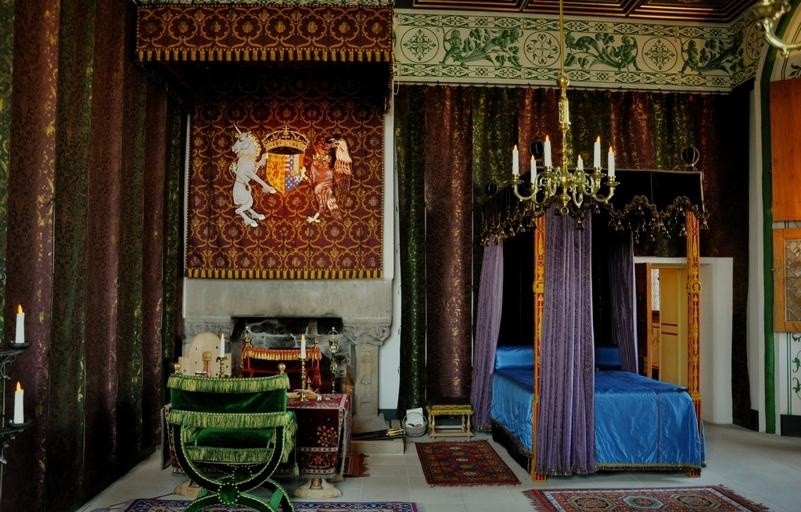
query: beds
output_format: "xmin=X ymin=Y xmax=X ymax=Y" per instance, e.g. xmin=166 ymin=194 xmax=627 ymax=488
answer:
xmin=467 ymin=131 xmax=712 ymax=482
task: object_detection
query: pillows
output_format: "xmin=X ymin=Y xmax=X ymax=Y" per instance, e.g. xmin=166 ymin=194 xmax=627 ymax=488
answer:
xmin=491 ymin=343 xmax=537 ymax=372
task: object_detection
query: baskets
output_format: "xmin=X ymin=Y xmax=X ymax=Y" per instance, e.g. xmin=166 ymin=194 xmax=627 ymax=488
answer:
xmin=402 ymin=412 xmax=428 ymax=437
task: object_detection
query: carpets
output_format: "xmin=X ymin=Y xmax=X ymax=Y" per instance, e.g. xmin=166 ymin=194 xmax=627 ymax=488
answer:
xmin=414 ymin=439 xmax=521 ymax=488
xmin=521 ymin=482 xmax=770 ymax=512
xmin=116 ymin=498 xmax=420 ymax=512
xmin=342 ymin=451 xmax=370 ymax=477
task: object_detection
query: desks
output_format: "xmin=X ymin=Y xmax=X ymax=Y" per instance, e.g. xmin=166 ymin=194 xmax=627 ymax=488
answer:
xmin=159 ymin=392 xmax=351 ymax=499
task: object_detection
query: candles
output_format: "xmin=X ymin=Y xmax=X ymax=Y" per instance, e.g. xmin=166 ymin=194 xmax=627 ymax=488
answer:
xmin=14 ymin=303 xmax=25 ymax=344
xmin=511 ymin=130 xmax=617 ymax=188
xmin=219 ymin=333 xmax=224 ymax=357
xmin=300 ymin=334 xmax=306 ymax=359
xmin=12 ymin=381 xmax=25 ymax=424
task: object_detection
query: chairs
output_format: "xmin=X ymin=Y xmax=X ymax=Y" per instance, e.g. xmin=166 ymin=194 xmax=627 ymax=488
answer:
xmin=165 ymin=361 xmax=297 ymax=512
xmin=240 ymin=334 xmax=322 ymax=393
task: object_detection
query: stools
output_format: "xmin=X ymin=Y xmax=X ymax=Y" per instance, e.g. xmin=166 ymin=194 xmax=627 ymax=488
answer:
xmin=425 ymin=404 xmax=475 ymax=441
